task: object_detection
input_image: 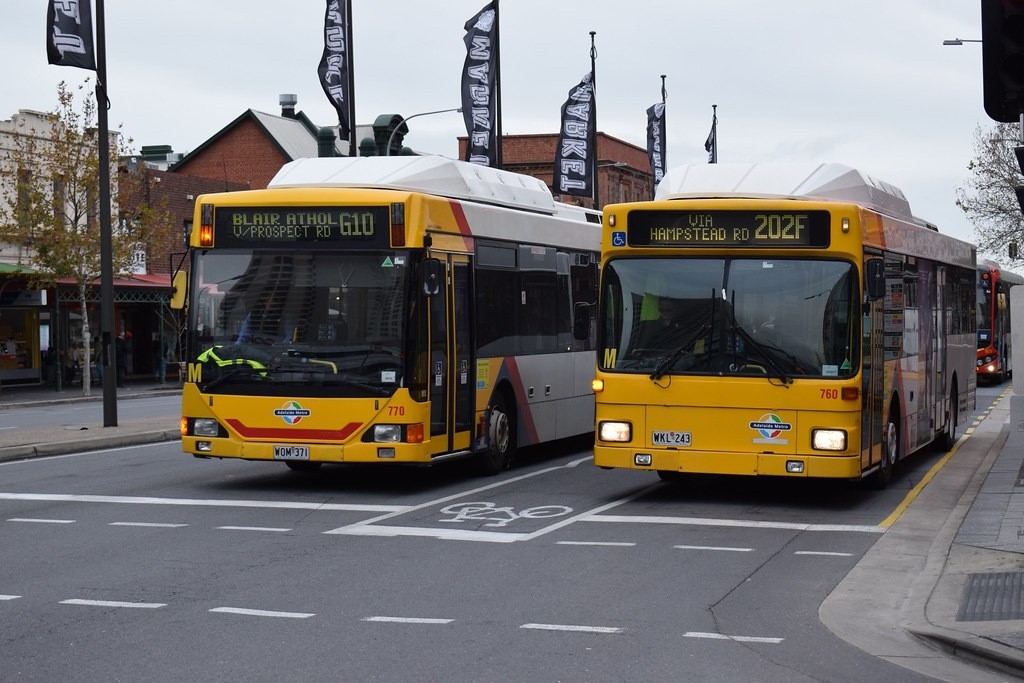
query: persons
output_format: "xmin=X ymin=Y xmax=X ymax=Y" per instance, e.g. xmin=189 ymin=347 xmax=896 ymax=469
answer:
xmin=116 ymin=330 xmax=126 ymax=387
xmin=236 ymin=286 xmax=293 ymax=362
xmin=67 ymin=344 xmax=80 ymax=383
xmin=91 ymin=337 xmax=104 ymax=385
xmin=632 ymin=295 xmax=701 ymax=360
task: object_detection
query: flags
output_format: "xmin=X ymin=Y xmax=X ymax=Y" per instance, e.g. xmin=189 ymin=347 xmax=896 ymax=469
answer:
xmin=552 ymin=72 xmax=593 ymax=198
xmin=46 ymin=0 xmax=96 ymax=71
xmin=705 ymin=120 xmax=716 ymax=163
xmin=461 ymin=0 xmax=498 ymax=168
xmin=318 ymin=0 xmax=349 ymax=141
xmin=646 ymin=103 xmax=664 ymax=197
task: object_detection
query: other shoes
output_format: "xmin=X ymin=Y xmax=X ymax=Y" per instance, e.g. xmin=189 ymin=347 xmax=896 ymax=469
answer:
xmin=117 ymin=383 xmax=125 ymax=388
xmin=161 ymin=381 xmax=167 ymax=384
xmin=155 ymin=376 xmax=159 ymax=382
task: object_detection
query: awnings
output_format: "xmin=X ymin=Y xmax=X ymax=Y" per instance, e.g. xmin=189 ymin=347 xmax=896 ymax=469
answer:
xmin=38 ymin=274 xmax=176 ymax=289
xmin=0 ymin=264 xmax=49 ymax=291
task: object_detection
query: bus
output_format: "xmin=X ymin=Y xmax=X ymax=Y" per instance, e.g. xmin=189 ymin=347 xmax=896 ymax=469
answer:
xmin=169 ymin=155 xmax=603 ymax=475
xmin=573 ymin=162 xmax=978 ymax=491
xmin=976 ymin=259 xmax=1024 ymax=385
